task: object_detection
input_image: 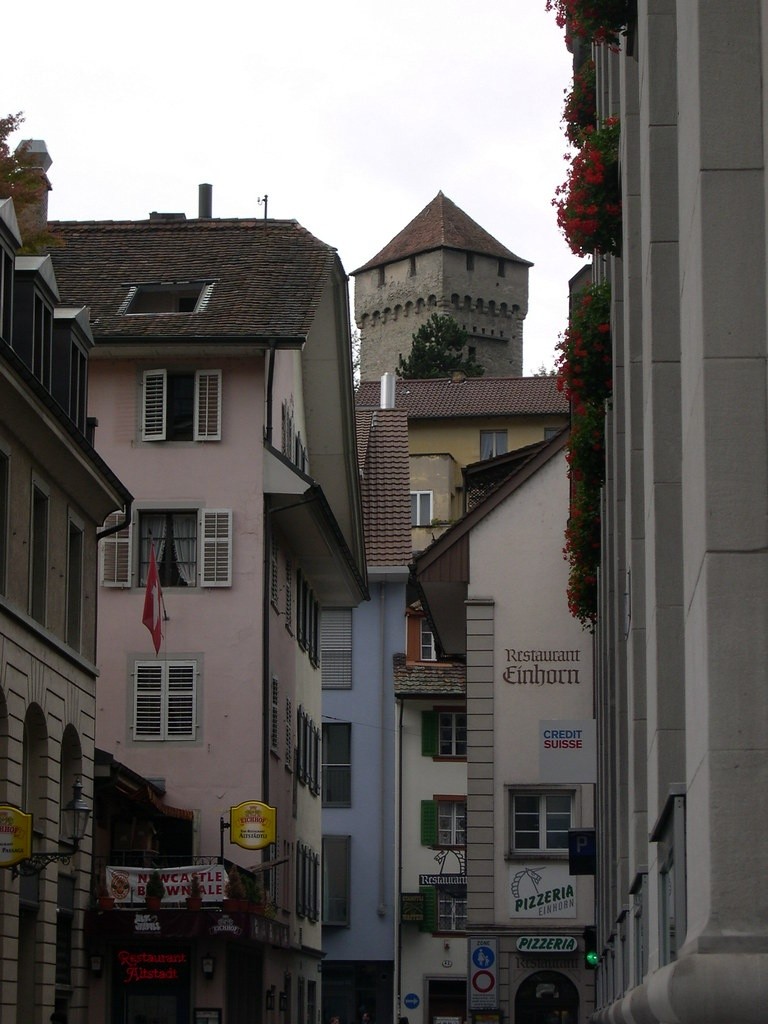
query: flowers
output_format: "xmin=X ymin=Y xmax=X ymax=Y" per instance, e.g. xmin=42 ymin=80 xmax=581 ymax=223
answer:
xmin=545 ymin=0 xmax=637 ymax=633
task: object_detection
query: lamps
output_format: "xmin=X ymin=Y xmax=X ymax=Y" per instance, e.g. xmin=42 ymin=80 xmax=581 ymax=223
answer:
xmin=285 ymin=965 xmax=291 ymax=985
xmin=444 ymin=939 xmax=449 ymax=950
xmin=266 ymin=983 xmax=276 ymax=1010
xmin=88 ymin=952 xmax=104 ymax=978
xmin=201 ymin=952 xmax=215 ymax=981
xmin=279 ymin=991 xmax=289 ymax=1011
xmin=11 ymin=776 xmax=93 ymax=882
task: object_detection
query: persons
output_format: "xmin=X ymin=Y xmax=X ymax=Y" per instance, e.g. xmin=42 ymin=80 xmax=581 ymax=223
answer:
xmin=360 ymin=1012 xmax=373 ymax=1024
xmin=329 ymin=1016 xmax=342 ymax=1024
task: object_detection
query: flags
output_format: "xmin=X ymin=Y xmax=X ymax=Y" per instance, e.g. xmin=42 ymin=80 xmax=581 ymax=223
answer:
xmin=142 ymin=544 xmax=162 ymax=657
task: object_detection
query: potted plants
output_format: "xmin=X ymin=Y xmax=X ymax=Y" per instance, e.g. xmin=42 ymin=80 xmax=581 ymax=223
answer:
xmin=223 ymin=863 xmax=266 ymax=914
xmin=95 ymin=884 xmax=115 ymax=909
xmin=144 ymin=871 xmax=165 ymax=912
xmin=186 ymin=880 xmax=202 ymax=912
